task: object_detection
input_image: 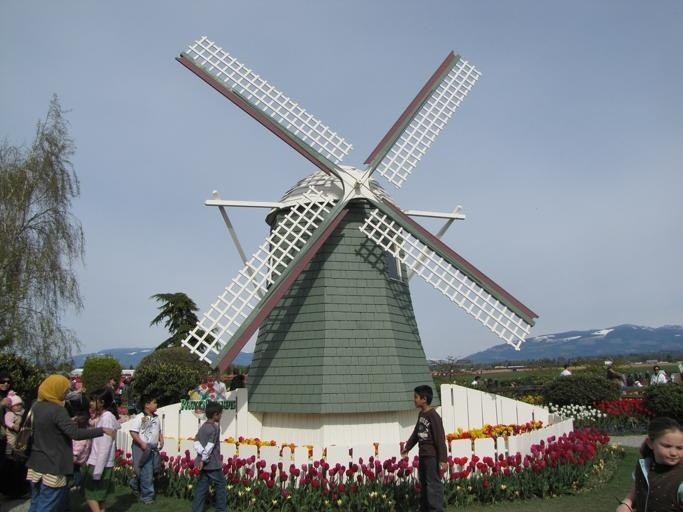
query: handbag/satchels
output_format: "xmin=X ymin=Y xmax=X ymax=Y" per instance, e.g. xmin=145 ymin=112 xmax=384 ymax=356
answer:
xmin=12 ymin=402 xmax=34 ymax=463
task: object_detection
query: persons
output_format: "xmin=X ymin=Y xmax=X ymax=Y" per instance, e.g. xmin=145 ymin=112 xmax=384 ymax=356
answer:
xmin=613 ymin=416 xmax=683 ymax=512
xmin=400 ymin=385 xmax=449 ymax=512
xmin=0 ymin=366 xmax=247 ymax=511
xmin=469 ymin=361 xmax=681 ymax=389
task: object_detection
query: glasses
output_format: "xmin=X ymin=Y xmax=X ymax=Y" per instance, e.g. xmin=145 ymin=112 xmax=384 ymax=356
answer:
xmin=0 ymin=379 xmax=10 ymax=384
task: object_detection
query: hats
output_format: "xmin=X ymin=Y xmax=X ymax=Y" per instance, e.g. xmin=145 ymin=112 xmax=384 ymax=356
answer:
xmin=6 ymin=390 xmax=24 ymax=408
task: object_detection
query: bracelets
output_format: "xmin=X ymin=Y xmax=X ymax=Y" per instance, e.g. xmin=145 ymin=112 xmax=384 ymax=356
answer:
xmin=620 ymin=500 xmax=634 ymax=510
